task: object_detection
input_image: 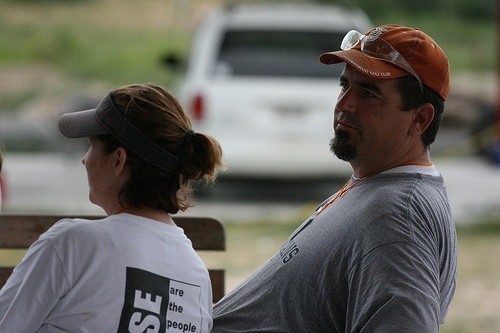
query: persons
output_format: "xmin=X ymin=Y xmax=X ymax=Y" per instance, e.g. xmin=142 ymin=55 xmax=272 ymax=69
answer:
xmin=0 ymin=84 xmax=223 ymax=333
xmin=210 ymin=23 xmax=458 ymax=333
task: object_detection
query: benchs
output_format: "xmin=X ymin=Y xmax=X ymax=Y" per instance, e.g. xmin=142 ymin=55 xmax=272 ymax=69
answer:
xmin=0 ymin=215 xmax=227 ymax=308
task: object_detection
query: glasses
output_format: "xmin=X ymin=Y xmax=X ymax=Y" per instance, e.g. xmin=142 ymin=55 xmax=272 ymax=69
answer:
xmin=340 ymin=30 xmax=426 ymax=104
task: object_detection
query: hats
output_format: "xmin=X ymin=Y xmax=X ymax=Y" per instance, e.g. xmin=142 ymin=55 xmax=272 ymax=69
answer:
xmin=319 ymin=24 xmax=450 ymax=100
xmin=58 ymin=93 xmax=183 ymax=176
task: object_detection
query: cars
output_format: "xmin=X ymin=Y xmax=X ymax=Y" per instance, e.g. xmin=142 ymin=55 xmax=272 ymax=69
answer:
xmin=158 ymin=0 xmax=375 ymax=195
